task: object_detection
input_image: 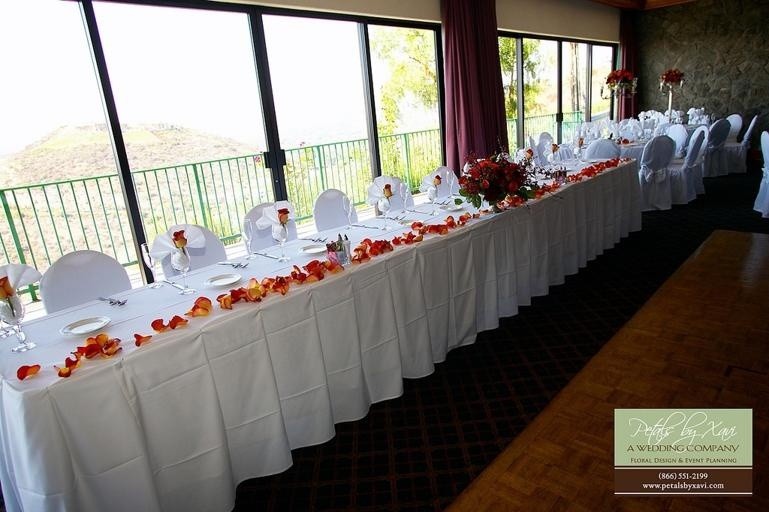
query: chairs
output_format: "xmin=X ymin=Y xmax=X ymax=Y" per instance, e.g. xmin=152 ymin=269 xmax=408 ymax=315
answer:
xmin=313 ymin=188 xmax=357 ymax=230
xmin=528 ymin=108 xmax=769 ymax=219
xmin=148 ymin=223 xmax=228 ymax=279
xmin=38 ymin=250 xmax=132 ymax=314
xmin=431 ymin=167 xmax=460 ymax=201
xmin=243 ymin=202 xmax=297 ymax=254
xmin=369 ymin=173 xmax=413 ymax=217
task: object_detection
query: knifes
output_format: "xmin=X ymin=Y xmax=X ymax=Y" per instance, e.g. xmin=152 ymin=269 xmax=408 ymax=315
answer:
xmin=163 ymin=279 xmax=186 ymax=290
xmin=252 ymin=250 xmax=280 ymax=260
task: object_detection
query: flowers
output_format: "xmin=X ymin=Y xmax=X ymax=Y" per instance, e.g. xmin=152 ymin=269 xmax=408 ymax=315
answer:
xmin=605 ymin=69 xmax=635 ymax=92
xmin=383 ymin=184 xmax=393 ymax=207
xmin=432 ymin=175 xmax=441 ymax=197
xmin=278 ymin=208 xmax=290 ymax=232
xmin=0 ymin=277 xmax=16 ymax=317
xmin=453 ymin=157 xmax=535 ymax=208
xmin=659 ymin=68 xmax=686 ymax=87
xmin=172 ymin=229 xmax=187 ymax=254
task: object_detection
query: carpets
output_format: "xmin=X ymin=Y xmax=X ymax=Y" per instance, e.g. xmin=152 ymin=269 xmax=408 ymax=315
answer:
xmin=232 ymin=169 xmax=769 ymax=512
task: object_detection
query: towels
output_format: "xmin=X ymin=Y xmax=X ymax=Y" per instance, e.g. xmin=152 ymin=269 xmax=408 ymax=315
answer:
xmin=367 ymin=174 xmax=402 ymax=213
xmin=256 ymin=201 xmax=294 ymax=246
xmin=0 ymin=264 xmax=41 ymax=300
xmin=419 ymin=167 xmax=455 ymax=204
xmin=150 ymin=225 xmax=206 ymax=274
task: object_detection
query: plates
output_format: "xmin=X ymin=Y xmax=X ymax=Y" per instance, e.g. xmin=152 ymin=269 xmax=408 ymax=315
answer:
xmin=59 ymin=314 xmax=109 ymax=337
xmin=300 ymin=244 xmax=330 ymax=256
xmin=203 ymin=273 xmax=244 ymax=287
xmin=439 ymin=201 xmax=464 ymax=212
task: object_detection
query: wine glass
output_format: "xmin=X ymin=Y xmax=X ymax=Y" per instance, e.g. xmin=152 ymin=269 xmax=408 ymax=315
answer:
xmin=514 ymin=145 xmax=600 ymax=182
xmin=342 ymin=171 xmax=458 ymax=233
xmin=172 ymin=246 xmax=201 ymax=297
xmin=270 ymin=224 xmax=292 ymax=263
xmin=140 ymin=241 xmax=166 ymax=291
xmin=242 ymin=219 xmax=257 ymax=262
xmin=0 ymin=294 xmax=36 ymax=353
